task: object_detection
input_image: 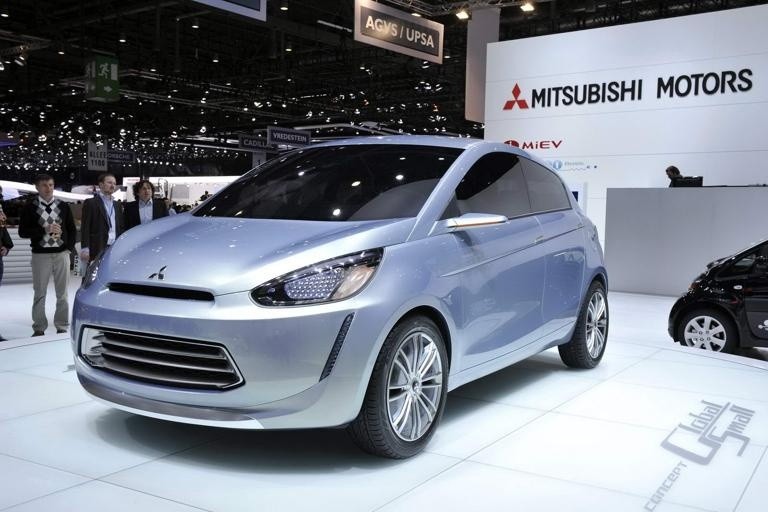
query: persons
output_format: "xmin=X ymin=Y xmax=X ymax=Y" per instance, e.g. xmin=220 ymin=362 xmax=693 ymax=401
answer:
xmin=173 ymin=190 xmax=209 ymax=214
xmin=665 ymin=165 xmax=683 ymax=187
xmin=0 ymin=173 xmax=78 ymax=342
xmin=80 ymin=173 xmax=177 ymax=285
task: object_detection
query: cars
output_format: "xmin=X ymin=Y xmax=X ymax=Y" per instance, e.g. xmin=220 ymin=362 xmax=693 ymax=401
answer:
xmin=66 ymin=125 xmax=609 ymax=464
xmin=664 ymin=237 xmax=768 ymax=360
xmin=3 ymin=196 xmax=33 ymax=226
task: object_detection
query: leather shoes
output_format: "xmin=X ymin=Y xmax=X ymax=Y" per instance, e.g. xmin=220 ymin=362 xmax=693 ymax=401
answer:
xmin=32 ymin=330 xmax=67 ymax=336
